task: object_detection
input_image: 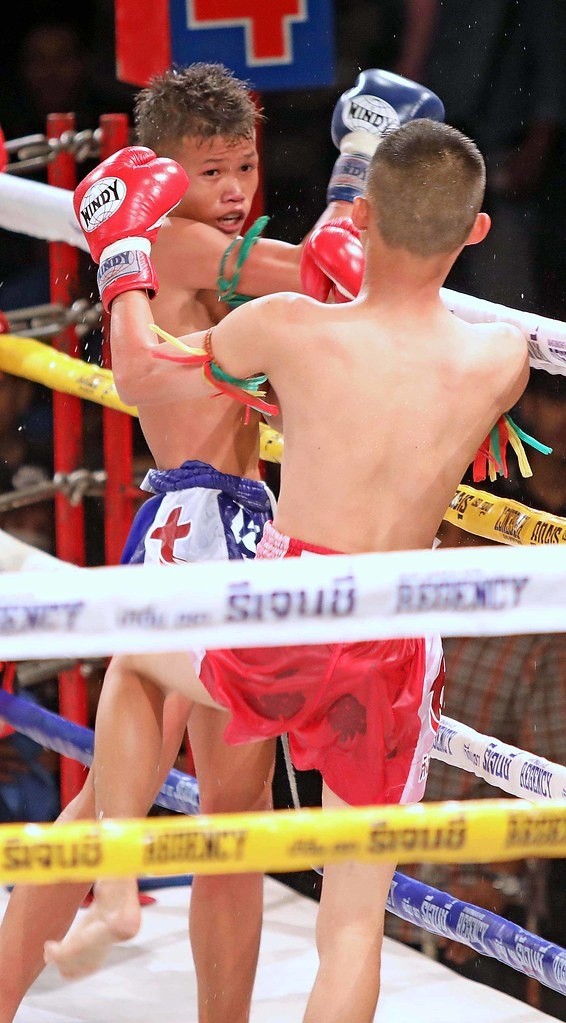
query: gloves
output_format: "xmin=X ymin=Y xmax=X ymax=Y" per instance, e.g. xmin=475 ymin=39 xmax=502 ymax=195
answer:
xmin=326 ymin=69 xmax=444 ymax=205
xmin=73 ymin=146 xmax=190 ymax=315
xmin=300 ymin=217 xmax=364 ymax=305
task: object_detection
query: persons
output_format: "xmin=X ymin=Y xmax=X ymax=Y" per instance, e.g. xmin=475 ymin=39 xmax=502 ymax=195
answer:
xmin=0 ymin=64 xmax=447 ymax=1023
xmin=0 ymin=0 xmax=566 ymax=998
xmin=73 ymin=116 xmax=530 ymax=1023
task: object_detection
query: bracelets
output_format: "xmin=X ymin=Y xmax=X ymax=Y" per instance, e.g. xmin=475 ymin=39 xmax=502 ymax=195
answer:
xmin=478 ymin=869 xmax=522 ymax=897
